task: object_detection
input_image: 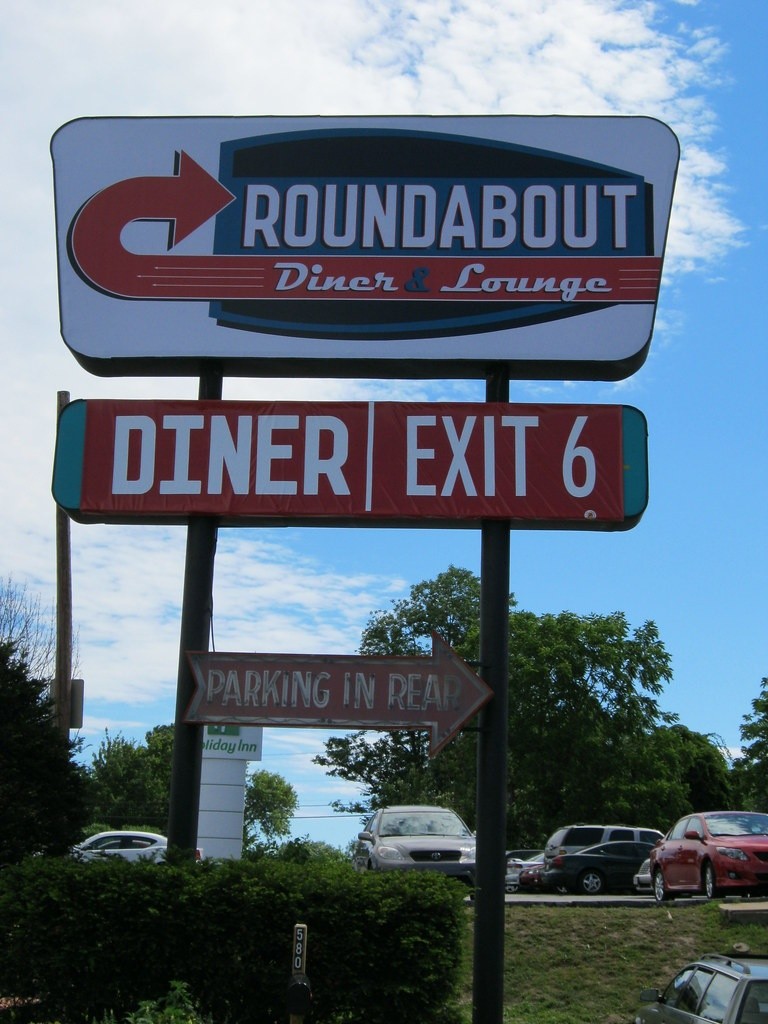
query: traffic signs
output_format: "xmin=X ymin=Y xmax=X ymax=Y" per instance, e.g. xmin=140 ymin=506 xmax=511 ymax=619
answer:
xmin=182 ymin=624 xmax=495 ymax=763
xmin=47 ymin=112 xmax=684 ymax=382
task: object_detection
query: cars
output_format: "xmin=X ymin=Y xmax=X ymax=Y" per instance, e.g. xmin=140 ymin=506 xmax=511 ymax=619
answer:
xmin=502 ymin=824 xmax=665 ymax=896
xmin=355 ymin=802 xmax=476 ymax=901
xmin=650 ymin=809 xmax=768 ymax=903
xmin=625 ymin=952 xmax=768 ymax=1024
xmin=34 ymin=828 xmax=202 ymax=871
